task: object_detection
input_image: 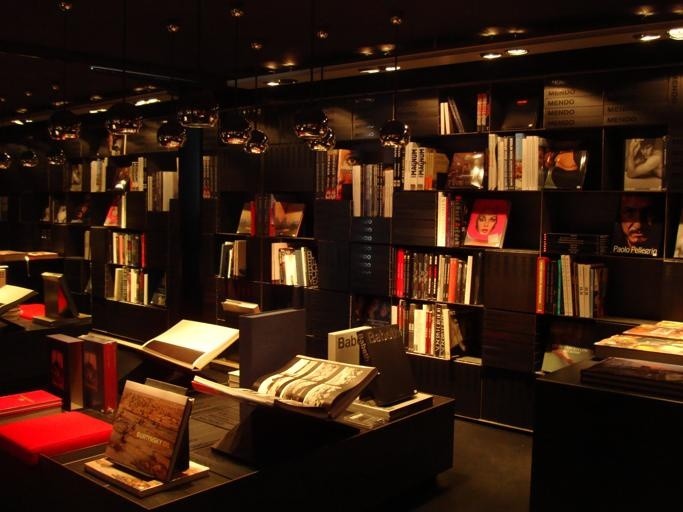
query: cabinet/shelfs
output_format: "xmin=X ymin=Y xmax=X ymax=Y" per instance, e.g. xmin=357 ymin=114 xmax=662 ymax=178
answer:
xmin=0 ymin=39 xmax=683 ymax=433
